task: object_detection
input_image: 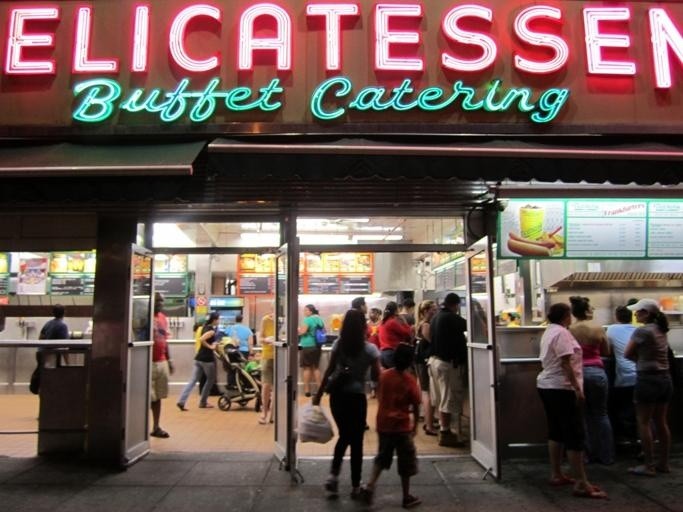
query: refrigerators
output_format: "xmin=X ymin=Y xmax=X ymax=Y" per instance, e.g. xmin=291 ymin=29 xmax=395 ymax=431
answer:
xmin=208 ymin=296 xmax=244 ymax=334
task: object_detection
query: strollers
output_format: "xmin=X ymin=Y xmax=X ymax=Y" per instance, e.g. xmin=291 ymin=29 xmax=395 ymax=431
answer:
xmin=211 ymin=341 xmax=263 ymax=411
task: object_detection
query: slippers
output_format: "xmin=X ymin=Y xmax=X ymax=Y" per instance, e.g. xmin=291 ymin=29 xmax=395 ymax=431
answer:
xmin=151 ymin=429 xmax=169 ymax=438
xmin=259 ymin=414 xmax=267 ymax=424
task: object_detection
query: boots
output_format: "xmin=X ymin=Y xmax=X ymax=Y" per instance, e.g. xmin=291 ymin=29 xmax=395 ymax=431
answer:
xmin=440 ymin=430 xmax=465 ymax=448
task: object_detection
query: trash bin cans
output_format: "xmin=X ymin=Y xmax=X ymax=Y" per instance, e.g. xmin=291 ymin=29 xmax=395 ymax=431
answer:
xmin=37 ymin=345 xmax=94 ymax=456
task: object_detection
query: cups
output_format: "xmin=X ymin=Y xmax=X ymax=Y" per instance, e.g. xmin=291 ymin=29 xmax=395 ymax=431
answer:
xmin=197 ymin=279 xmax=205 ymax=295
xmin=371 ymin=325 xmax=376 ymax=333
xmin=519 ymin=206 xmax=544 ymax=240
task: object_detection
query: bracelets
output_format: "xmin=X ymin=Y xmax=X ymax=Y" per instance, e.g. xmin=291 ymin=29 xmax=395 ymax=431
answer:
xmin=168 ymin=359 xmax=172 ymax=362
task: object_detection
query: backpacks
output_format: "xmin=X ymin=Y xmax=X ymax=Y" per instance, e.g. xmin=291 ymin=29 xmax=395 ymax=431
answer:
xmin=308 ymin=317 xmax=327 ymax=345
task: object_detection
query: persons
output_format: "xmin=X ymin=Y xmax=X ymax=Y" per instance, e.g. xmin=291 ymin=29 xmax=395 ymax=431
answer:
xmin=605 ymin=305 xmax=646 ymax=460
xmin=625 ymin=298 xmax=646 ymax=327
xmin=472 ymin=299 xmax=488 ymax=343
xmin=506 ymin=313 xmax=521 ymax=327
xmin=151 ymin=293 xmax=174 ymax=438
xmin=38 ymin=305 xmax=71 ymax=367
xmin=537 ymin=303 xmax=608 ymax=499
xmin=623 ymin=298 xmax=674 ymax=477
xmin=569 ymin=296 xmax=617 ymax=465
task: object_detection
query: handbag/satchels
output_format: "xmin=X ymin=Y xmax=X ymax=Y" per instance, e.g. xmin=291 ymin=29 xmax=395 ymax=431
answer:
xmin=414 ymin=339 xmax=431 ymax=357
xmin=325 ymin=368 xmax=352 ymax=393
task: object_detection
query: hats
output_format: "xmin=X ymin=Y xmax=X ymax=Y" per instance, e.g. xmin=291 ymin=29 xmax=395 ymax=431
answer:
xmin=627 ymin=299 xmax=659 ymax=313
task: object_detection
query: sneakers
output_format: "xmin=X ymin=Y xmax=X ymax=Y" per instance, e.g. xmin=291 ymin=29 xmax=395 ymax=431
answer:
xmin=572 ymin=480 xmax=608 ymax=498
xmin=403 ymin=494 xmax=419 ymax=508
xmin=657 ymin=464 xmax=671 ymax=473
xmin=419 ymin=414 xmax=439 ymax=436
xmin=627 ymin=463 xmax=656 ymax=476
xmin=548 ymin=475 xmax=574 ymax=487
xmin=177 ymin=402 xmax=187 ymax=411
xmin=325 ymin=480 xmax=338 ymax=493
xmin=200 ymin=405 xmax=213 ymax=408
xmin=350 ymin=489 xmax=373 ymax=505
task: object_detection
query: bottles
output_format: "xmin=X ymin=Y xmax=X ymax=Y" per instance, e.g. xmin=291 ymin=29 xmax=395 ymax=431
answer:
xmin=229 ymin=280 xmax=236 ymax=295
xmin=223 ymin=271 xmax=229 ymax=294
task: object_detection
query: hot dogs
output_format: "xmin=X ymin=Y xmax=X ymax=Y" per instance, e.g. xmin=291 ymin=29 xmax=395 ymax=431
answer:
xmin=507 ymin=234 xmax=557 ymax=257
